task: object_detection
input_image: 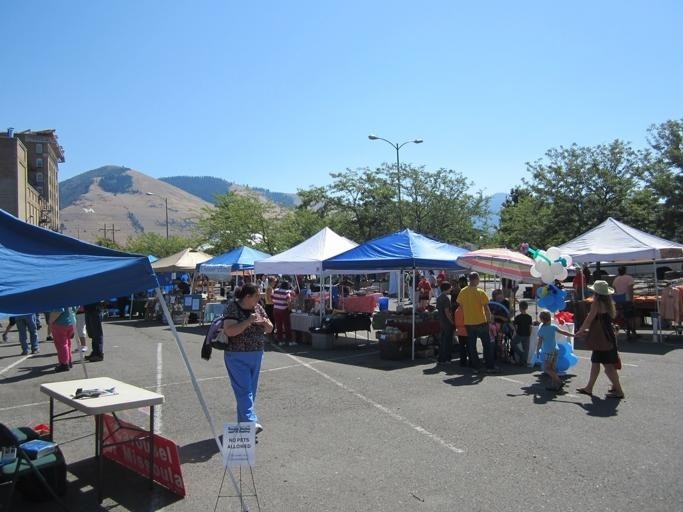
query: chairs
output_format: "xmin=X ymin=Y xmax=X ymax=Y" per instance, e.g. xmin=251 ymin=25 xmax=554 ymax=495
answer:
xmin=0 ymin=421 xmax=63 ymax=508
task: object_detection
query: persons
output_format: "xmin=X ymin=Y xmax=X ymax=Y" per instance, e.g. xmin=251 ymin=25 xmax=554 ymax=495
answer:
xmin=536 ymin=311 xmax=584 ymax=391
xmin=2 ymin=316 xmax=16 ymax=342
xmin=488 ymin=288 xmax=518 ymax=365
xmin=612 ymin=266 xmax=643 ymax=340
xmin=74 ymin=304 xmax=88 ymax=352
xmin=224 ymin=282 xmax=274 ymax=441
xmin=450 ymin=279 xmax=459 ymax=322
xmin=48 ymin=305 xmax=80 ymax=371
xmin=175 ymin=277 xmax=190 ymax=297
xmin=456 ymin=271 xmax=503 ymax=374
xmin=489 ymin=313 xmax=497 ymax=364
xmin=15 ymin=313 xmax=40 ymax=355
xmin=405 ymin=269 xmax=447 ymax=311
xmin=454 ymin=303 xmax=470 ymax=367
xmin=44 ymin=311 xmax=54 ymax=341
xmin=458 ymin=276 xmax=468 ymax=289
xmin=225 ymin=269 xmax=267 ymax=285
xmin=510 ymin=300 xmax=532 ymax=368
xmin=35 ymin=312 xmax=42 ymax=330
xmin=436 ymin=281 xmax=456 ymax=365
xmin=574 ymin=280 xmax=625 ymax=398
xmin=265 ymin=277 xmax=277 ymax=339
xmin=271 ymin=280 xmax=300 ymax=347
xmin=553 ymin=278 xmax=562 ymax=291
xmin=83 ymin=300 xmax=106 ymax=361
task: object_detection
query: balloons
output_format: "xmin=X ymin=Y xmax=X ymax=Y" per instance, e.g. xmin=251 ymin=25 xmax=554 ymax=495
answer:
xmin=535 ymin=283 xmax=567 ymax=313
xmin=536 ymin=340 xmax=578 ymax=374
xmin=529 ymin=246 xmax=573 ymax=285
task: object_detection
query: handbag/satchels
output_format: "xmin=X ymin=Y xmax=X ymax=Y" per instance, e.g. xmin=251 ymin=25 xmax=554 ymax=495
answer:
xmin=588 ymin=319 xmax=610 ymax=351
xmin=201 ymin=316 xmax=229 ymax=360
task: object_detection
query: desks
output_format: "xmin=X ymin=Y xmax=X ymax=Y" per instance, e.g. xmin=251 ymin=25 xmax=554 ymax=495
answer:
xmin=39 ymin=376 xmax=165 ymax=507
xmin=339 ymin=292 xmax=383 ymax=315
xmin=372 ymin=311 xmax=443 ymax=357
xmin=585 ymin=295 xmax=665 ymax=341
xmin=290 ymin=312 xmax=370 ymax=341
xmin=204 ymin=303 xmax=228 ymax=327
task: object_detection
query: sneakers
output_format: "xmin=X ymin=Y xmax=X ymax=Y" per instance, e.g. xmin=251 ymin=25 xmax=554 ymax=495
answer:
xmin=55 ymin=364 xmax=70 ymax=372
xmin=47 ymin=336 xmax=53 ymax=340
xmin=89 ymin=353 xmax=103 ymax=362
xmin=32 ymin=349 xmax=40 ymax=354
xmin=21 ymin=352 xmax=28 ymax=355
xmin=255 ymin=423 xmax=263 ymax=434
xmin=85 ymin=353 xmax=93 ymax=360
xmin=3 ymin=334 xmax=8 ymax=343
xmin=82 ymin=346 xmax=87 ymax=352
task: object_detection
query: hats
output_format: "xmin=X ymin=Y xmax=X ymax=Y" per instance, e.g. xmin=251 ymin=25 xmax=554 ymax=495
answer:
xmin=587 ymin=280 xmax=614 ymax=295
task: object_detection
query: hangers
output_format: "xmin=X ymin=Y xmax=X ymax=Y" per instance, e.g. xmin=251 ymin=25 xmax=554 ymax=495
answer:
xmin=663 ymin=278 xmax=683 ymax=292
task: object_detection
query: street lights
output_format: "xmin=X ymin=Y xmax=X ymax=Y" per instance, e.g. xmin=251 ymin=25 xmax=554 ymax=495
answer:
xmin=366 ymin=134 xmax=423 ymax=233
xmin=145 ymin=192 xmax=168 ymax=244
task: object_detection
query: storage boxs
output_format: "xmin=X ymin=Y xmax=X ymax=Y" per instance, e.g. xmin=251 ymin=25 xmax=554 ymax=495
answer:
xmin=376 ymin=330 xmax=410 ymax=361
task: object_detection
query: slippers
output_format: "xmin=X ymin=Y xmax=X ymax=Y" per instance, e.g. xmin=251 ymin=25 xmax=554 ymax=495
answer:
xmin=576 ymin=387 xmax=592 ymax=395
xmin=605 ymin=392 xmax=624 ymax=398
